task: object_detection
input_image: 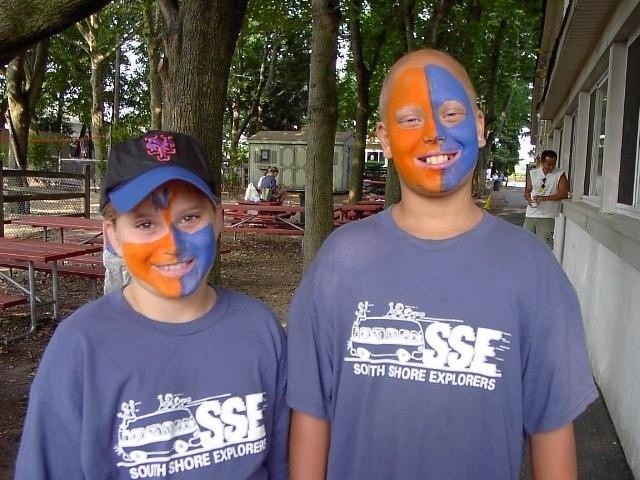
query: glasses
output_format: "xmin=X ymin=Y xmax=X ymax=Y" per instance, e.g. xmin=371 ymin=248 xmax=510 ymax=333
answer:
xmin=541 ymin=176 xmax=547 ymax=189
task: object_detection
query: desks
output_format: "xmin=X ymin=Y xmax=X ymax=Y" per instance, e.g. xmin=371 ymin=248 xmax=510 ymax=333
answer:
xmin=11 ymin=215 xmax=103 ymax=245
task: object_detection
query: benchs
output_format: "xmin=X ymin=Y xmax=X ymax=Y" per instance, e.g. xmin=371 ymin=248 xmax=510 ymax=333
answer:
xmin=1 ymin=238 xmax=108 ymax=347
xmin=217 ymin=168 xmax=387 ymax=245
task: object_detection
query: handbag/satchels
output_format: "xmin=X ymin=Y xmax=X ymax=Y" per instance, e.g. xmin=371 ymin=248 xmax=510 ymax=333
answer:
xmin=260 ymin=185 xmax=273 ymax=201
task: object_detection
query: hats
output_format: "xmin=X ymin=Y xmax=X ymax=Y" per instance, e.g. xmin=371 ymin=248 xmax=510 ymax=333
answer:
xmin=96 ymin=126 xmax=222 ymax=221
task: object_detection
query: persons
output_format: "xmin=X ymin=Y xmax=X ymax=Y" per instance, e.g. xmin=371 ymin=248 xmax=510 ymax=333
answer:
xmin=11 ymin=127 xmax=293 ymax=480
xmin=257 ymin=165 xmax=281 ymax=192
xmin=522 ymin=149 xmax=570 ymax=252
xmin=261 ymin=167 xmax=288 ymax=203
xmin=283 ymin=47 xmax=600 ymax=480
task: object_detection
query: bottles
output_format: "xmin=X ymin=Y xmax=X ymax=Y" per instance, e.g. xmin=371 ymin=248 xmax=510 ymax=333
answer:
xmin=530 ymin=188 xmax=538 ymax=207
